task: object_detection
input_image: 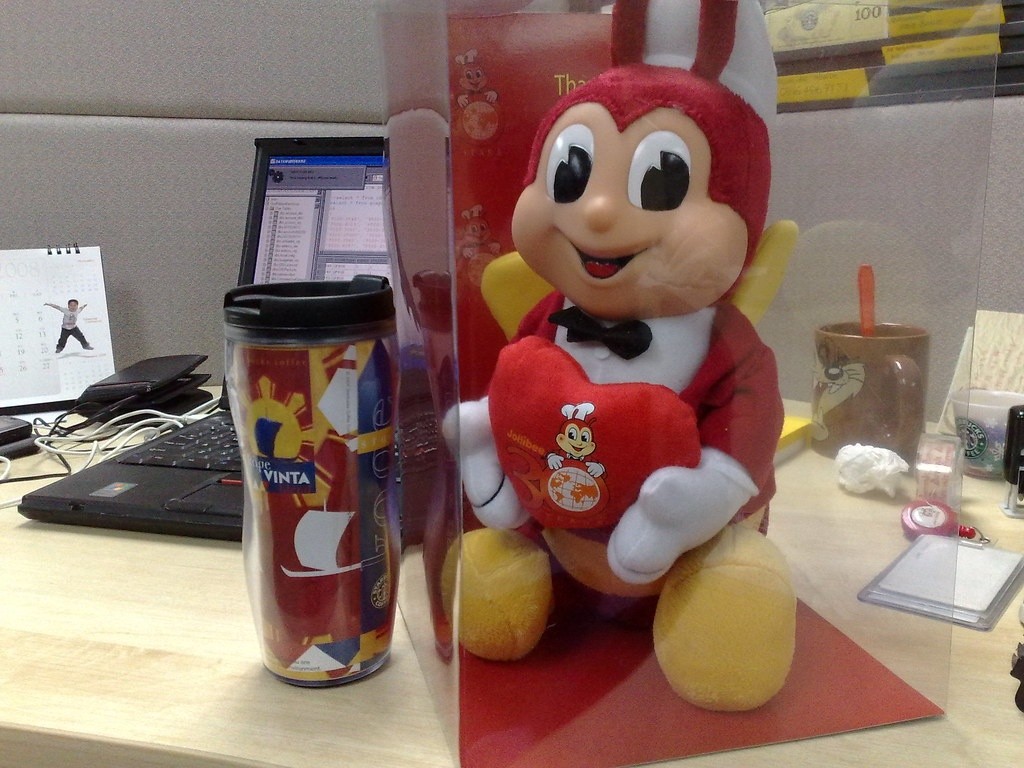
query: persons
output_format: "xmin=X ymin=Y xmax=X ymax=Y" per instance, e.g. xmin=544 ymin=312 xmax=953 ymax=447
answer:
xmin=44 ymin=299 xmax=94 ymax=353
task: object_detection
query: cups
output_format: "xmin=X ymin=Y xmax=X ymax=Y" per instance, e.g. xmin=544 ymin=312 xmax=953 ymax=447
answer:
xmin=812 ymin=323 xmax=931 ymax=462
xmin=950 ymin=390 xmax=1024 ymax=480
xmin=219 ymin=275 xmax=400 ymax=691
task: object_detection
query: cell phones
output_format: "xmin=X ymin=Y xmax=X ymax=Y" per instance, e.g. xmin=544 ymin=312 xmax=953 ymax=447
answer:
xmin=0 ymin=416 xmax=32 ymax=447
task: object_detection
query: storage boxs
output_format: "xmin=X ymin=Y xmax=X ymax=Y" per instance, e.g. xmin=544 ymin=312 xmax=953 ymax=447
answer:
xmin=376 ymin=0 xmax=1004 ymax=768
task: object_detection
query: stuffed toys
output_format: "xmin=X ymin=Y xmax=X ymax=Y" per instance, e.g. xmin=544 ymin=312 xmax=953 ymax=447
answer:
xmin=445 ymin=0 xmax=796 ymax=710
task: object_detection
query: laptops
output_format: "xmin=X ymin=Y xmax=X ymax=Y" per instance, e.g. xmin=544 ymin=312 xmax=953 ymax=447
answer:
xmin=15 ymin=136 xmax=388 ymax=540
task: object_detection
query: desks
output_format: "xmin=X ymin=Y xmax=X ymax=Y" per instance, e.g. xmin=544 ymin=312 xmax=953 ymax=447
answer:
xmin=0 ymin=385 xmax=1024 ymax=768
